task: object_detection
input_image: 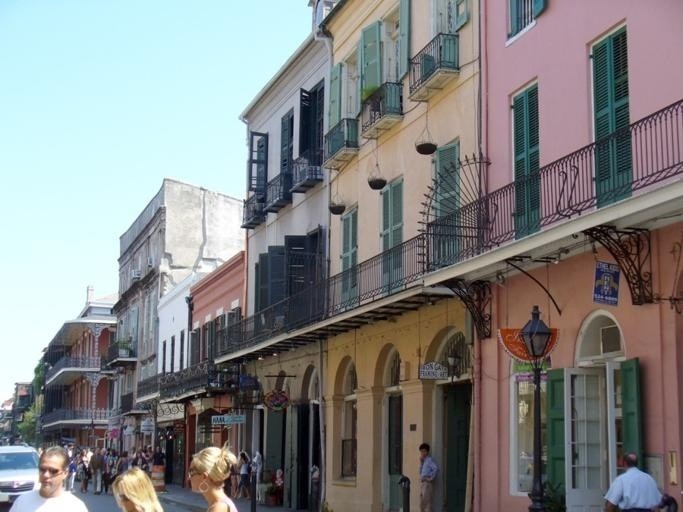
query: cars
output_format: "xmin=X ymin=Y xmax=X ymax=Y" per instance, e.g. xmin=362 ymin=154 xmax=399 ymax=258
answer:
xmin=0 ymin=444 xmax=42 ymax=506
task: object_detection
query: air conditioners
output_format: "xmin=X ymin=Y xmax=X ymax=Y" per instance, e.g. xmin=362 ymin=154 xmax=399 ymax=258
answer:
xmin=130 ymin=269 xmax=140 ymax=279
xmin=145 ymin=257 xmax=152 ymax=267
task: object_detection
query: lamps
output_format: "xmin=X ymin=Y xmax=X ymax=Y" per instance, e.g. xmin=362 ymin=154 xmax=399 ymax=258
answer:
xmin=445 ymin=348 xmax=461 ymax=384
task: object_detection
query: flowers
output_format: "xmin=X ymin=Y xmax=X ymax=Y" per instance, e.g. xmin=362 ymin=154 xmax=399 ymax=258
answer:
xmin=263 ymin=387 xmax=288 ymax=409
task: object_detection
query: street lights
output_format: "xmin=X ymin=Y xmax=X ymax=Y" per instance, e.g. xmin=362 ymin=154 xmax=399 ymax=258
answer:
xmin=519 ymin=304 xmax=553 ymax=512
xmin=118 ymin=416 xmax=125 ymax=455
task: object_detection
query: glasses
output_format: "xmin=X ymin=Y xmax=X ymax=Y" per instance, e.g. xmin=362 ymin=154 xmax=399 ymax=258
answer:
xmin=38 ymin=465 xmax=58 ymax=474
xmin=187 ymin=469 xmax=201 ymax=481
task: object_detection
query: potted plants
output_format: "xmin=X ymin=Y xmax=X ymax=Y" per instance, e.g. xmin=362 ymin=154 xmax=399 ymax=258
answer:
xmin=361 ymin=85 xmax=386 ymax=111
xmin=265 ymin=485 xmax=280 ymax=506
xmin=117 ymin=336 xmax=130 ymax=358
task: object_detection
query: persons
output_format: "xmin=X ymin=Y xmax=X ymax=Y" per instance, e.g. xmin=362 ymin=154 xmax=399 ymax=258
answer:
xmin=233 ymin=453 xmax=252 ymax=500
xmin=604 ymin=452 xmax=663 ymax=512
xmin=112 ymin=467 xmax=164 ymax=512
xmin=38 ymin=442 xmax=166 ymax=495
xmin=189 ymin=440 xmax=238 ymax=512
xmin=419 ymin=443 xmax=439 ymax=512
xmin=9 ymin=446 xmax=89 ymax=512
